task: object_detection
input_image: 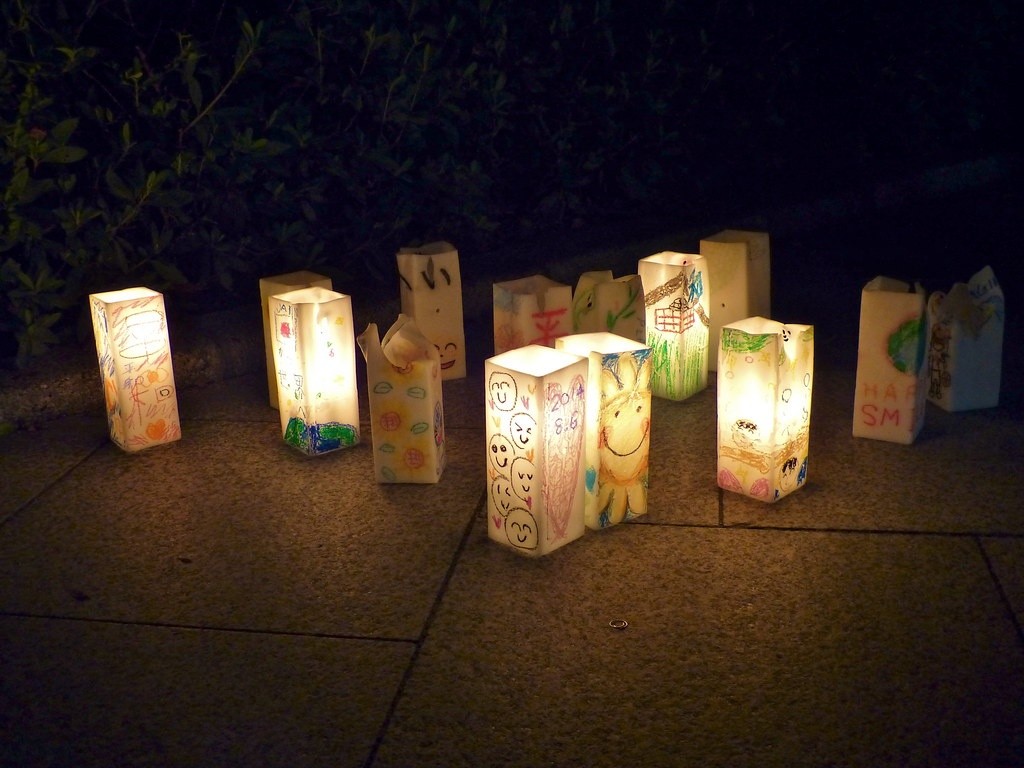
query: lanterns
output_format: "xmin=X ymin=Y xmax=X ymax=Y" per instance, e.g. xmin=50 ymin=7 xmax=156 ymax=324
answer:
xmin=852 ymin=275 xmax=929 ymax=445
xmin=90 ymin=285 xmax=182 ymax=451
xmin=267 ymin=285 xmax=360 ymax=457
xmin=484 ymin=344 xmax=589 ymax=557
xmin=356 ymin=312 xmax=449 ymax=484
xmin=718 ymin=315 xmax=813 ymax=503
xmin=638 ymin=250 xmax=711 ymax=401
xmin=261 ymin=270 xmax=333 ymax=411
xmin=925 ymin=262 xmax=1004 ymax=413
xmin=395 ymin=241 xmax=469 ymax=382
xmin=493 ymin=275 xmax=573 ymax=356
xmin=700 ymin=229 xmax=773 ymax=375
xmin=553 ymin=332 xmax=652 ymax=532
xmin=573 ymin=269 xmax=646 ymax=348
